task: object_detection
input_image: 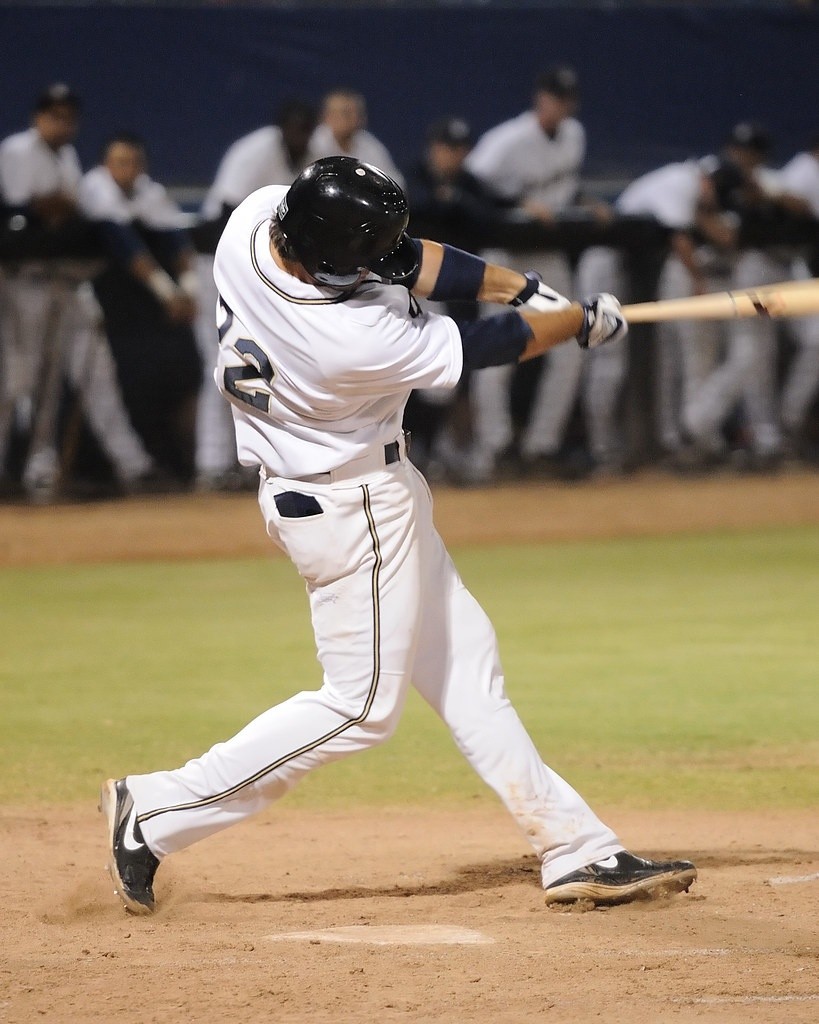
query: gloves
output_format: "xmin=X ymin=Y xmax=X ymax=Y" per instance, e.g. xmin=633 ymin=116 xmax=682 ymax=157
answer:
xmin=508 ymin=279 xmax=572 ymax=313
xmin=576 ymin=293 xmax=628 ymax=350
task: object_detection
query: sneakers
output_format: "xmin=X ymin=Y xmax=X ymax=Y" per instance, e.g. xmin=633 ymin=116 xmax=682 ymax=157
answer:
xmin=97 ymin=777 xmax=160 ymax=916
xmin=544 ymin=850 xmax=697 ymax=907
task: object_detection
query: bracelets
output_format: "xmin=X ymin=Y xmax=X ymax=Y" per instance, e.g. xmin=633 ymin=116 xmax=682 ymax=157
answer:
xmin=147 ymin=270 xmax=172 ymax=297
xmin=426 ymin=242 xmax=485 ymax=304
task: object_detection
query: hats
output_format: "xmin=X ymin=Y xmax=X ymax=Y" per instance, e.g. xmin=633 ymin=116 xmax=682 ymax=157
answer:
xmin=534 ymin=68 xmax=579 ymax=96
xmin=35 ymin=79 xmax=79 ymax=113
xmin=700 ymin=150 xmax=748 ymax=209
xmin=735 ymin=119 xmax=785 ymax=166
xmin=428 ymin=116 xmax=477 ymax=144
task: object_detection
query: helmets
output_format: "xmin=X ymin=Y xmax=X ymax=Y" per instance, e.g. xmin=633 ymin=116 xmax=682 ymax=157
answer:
xmin=277 ymin=156 xmax=419 ymax=287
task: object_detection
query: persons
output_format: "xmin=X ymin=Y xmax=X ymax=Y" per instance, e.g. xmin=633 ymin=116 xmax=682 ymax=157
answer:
xmin=99 ymin=155 xmax=697 ymax=915
xmin=0 ymin=67 xmax=819 ymax=490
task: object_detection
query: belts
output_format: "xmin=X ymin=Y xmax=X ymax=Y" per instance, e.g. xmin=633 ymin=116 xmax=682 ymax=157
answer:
xmin=319 ymin=429 xmax=412 ymax=474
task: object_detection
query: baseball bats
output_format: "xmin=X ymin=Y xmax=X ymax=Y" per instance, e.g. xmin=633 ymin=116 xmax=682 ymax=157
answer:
xmin=614 ymin=279 xmax=819 ymax=322
